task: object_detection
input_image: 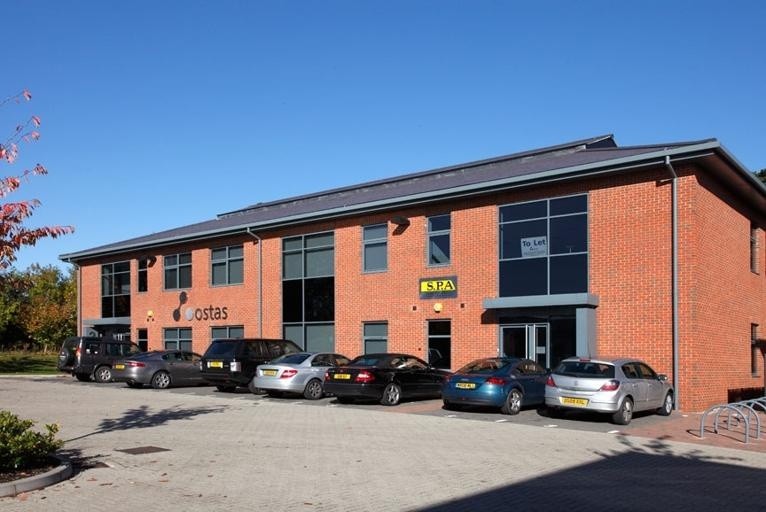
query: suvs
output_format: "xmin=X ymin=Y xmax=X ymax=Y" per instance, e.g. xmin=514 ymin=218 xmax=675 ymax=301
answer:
xmin=198 ymin=337 xmax=306 ymax=394
xmin=56 ymin=335 xmax=145 ymax=385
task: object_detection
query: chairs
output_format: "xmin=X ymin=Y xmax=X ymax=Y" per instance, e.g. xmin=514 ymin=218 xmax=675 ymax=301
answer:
xmin=587 ymin=363 xmax=636 ymax=377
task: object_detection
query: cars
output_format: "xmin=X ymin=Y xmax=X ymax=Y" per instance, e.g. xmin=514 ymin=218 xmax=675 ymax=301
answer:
xmin=254 ymin=352 xmax=353 ymax=401
xmin=442 ymin=356 xmax=551 ymax=416
xmin=545 ymin=355 xmax=674 ymax=425
xmin=113 ymin=348 xmax=209 ymax=390
xmin=323 ymin=351 xmax=452 ymax=406
